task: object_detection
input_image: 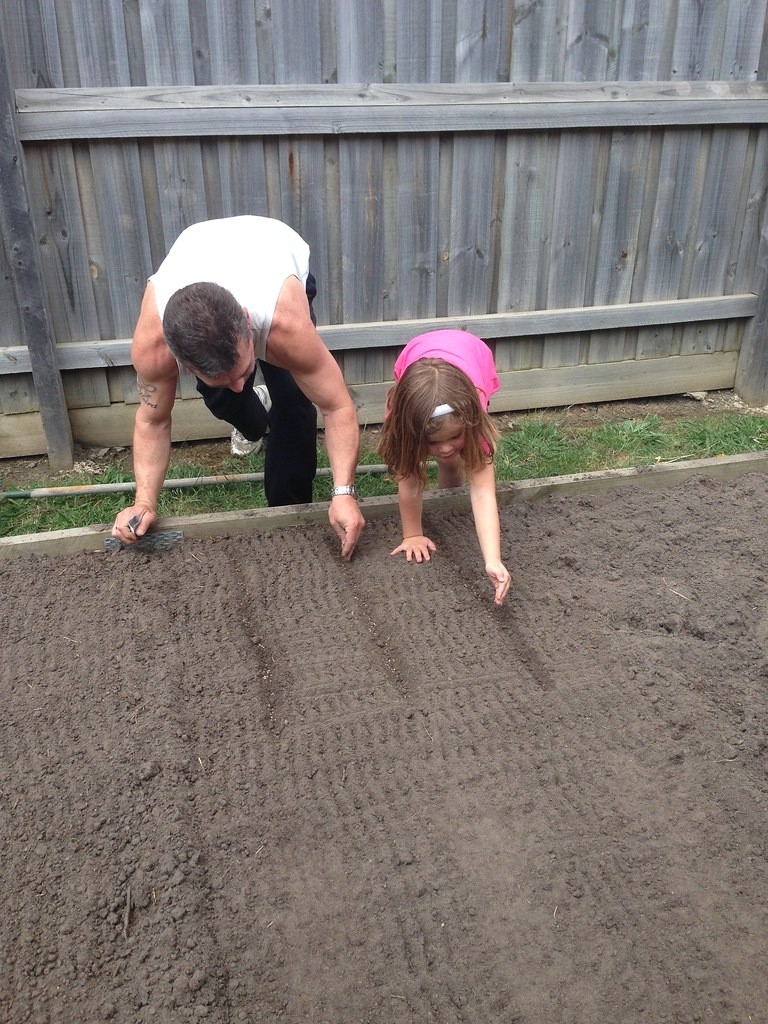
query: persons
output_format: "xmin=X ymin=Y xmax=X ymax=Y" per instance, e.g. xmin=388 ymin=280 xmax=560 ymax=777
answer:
xmin=111 ymin=215 xmax=365 ymax=563
xmin=377 ymin=329 xmax=511 ymax=605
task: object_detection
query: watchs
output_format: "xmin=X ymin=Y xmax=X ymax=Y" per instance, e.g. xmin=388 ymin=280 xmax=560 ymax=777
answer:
xmin=332 ymin=484 xmax=359 ymax=500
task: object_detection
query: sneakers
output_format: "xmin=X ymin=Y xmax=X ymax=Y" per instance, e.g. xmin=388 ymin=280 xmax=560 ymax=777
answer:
xmin=230 ymin=384 xmax=272 ymax=459
